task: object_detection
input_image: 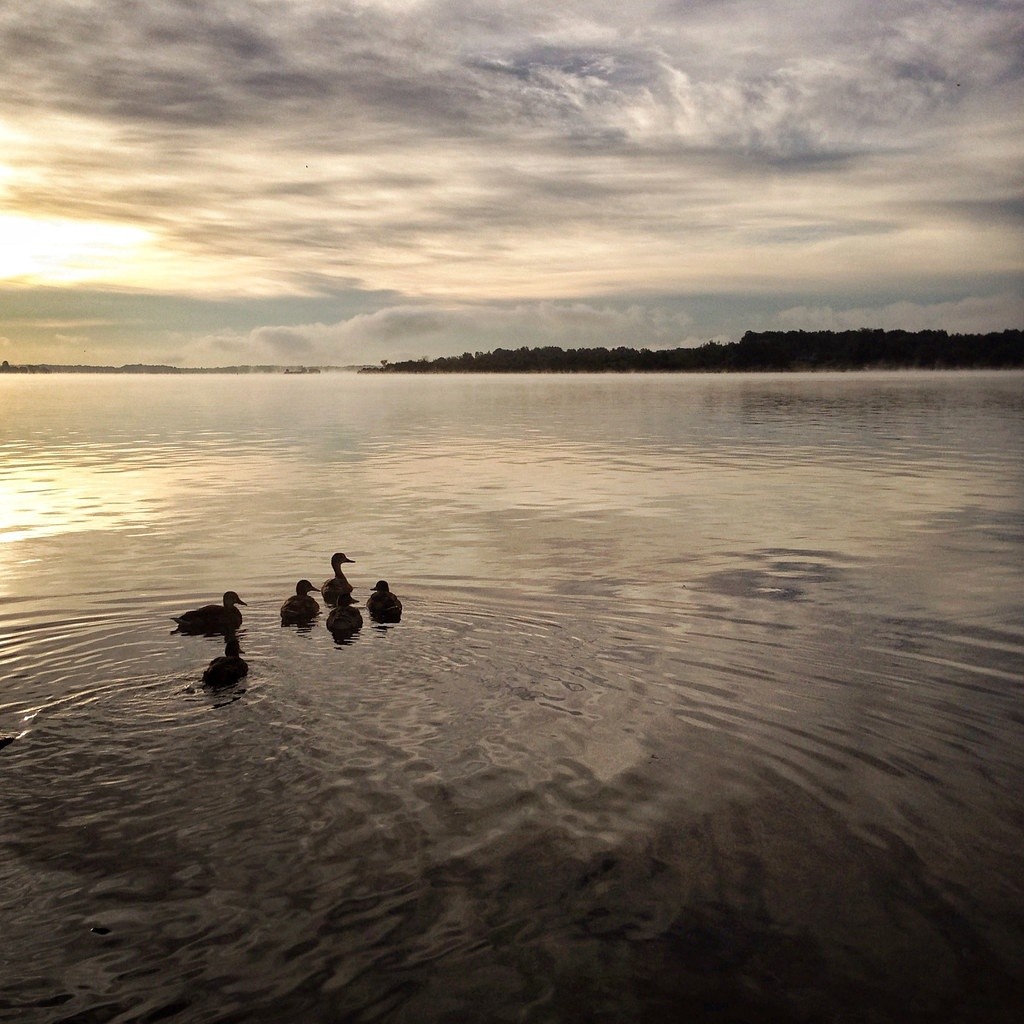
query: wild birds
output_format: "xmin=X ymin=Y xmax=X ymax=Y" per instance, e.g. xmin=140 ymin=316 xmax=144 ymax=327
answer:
xmin=366 ymin=580 xmax=403 ymax=624
xmin=280 ymin=579 xmax=322 ymax=625
xmin=322 ymin=553 xmax=364 ymax=634
xmin=170 ymin=591 xmax=249 ymax=689
